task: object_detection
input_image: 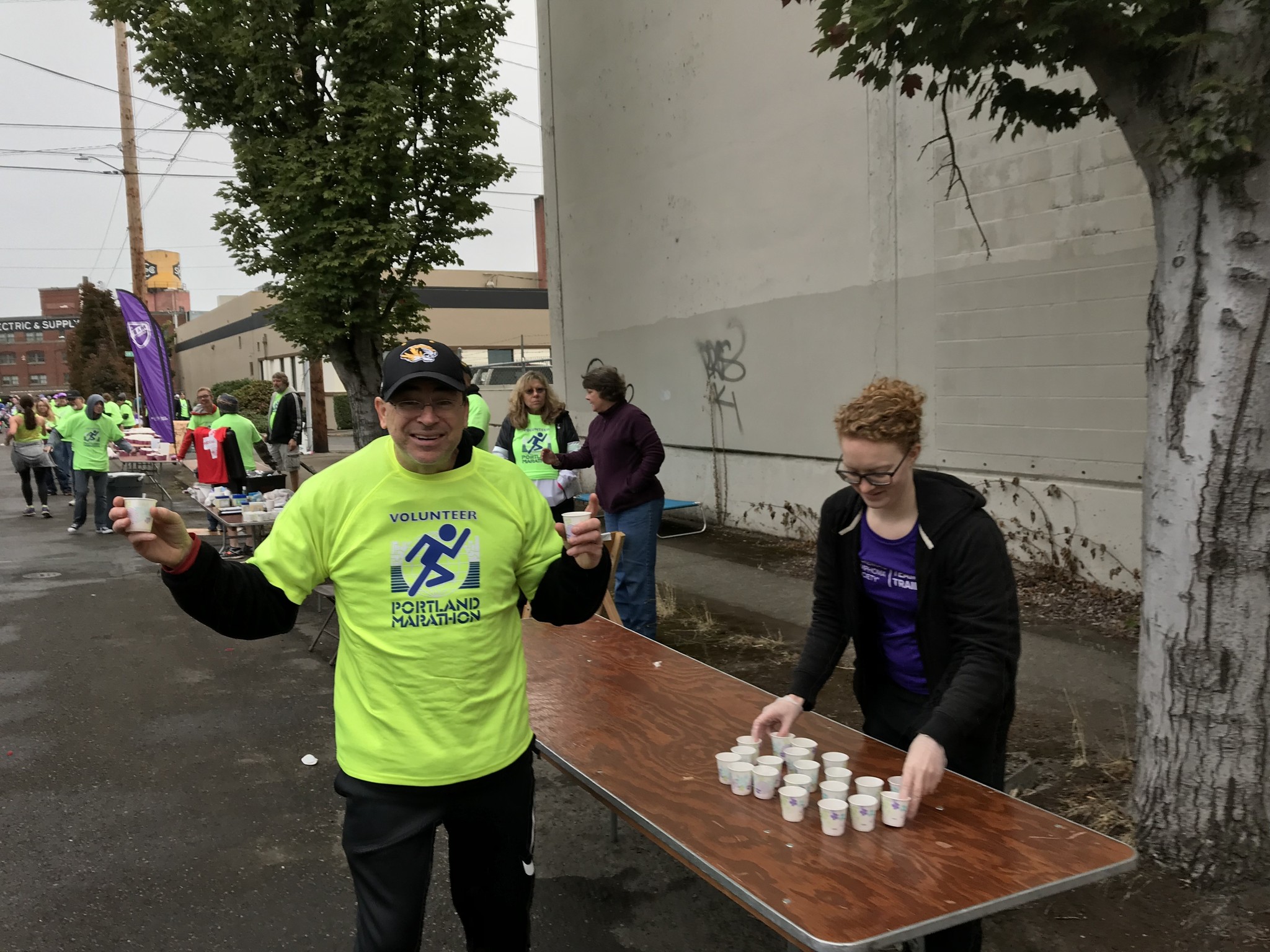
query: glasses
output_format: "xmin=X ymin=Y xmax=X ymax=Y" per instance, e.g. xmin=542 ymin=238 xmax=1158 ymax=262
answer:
xmin=524 ymin=387 xmax=546 ymax=394
xmin=834 ymin=438 xmax=914 ymax=486
xmin=67 ymin=398 xmax=76 ymax=401
xmin=387 ymin=400 xmax=465 ymax=416
xmin=197 ymin=395 xmax=210 ymax=399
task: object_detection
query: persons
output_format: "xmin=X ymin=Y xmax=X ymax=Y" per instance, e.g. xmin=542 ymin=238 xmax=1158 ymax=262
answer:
xmin=752 ymin=376 xmax=1023 ymax=952
xmin=493 ymin=364 xmax=667 ymax=641
xmin=107 ymin=338 xmax=613 ymax=952
xmin=266 ymin=371 xmax=303 ymax=493
xmin=0 ymin=391 xmax=149 ymax=534
xmin=459 ymin=360 xmax=492 ymax=453
xmin=172 ymin=387 xmax=281 ymax=561
xmin=173 ymin=391 xmax=193 ymax=419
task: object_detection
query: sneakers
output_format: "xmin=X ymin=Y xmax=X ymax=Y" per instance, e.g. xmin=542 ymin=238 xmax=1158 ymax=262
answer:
xmin=242 ymin=544 xmax=254 ymax=557
xmin=219 ymin=545 xmax=244 ymax=559
xmin=68 ymin=522 xmax=82 ymax=533
xmin=95 ymin=526 xmax=114 ymax=534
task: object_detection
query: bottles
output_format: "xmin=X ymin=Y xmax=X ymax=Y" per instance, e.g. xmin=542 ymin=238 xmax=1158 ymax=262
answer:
xmin=242 ymin=486 xmax=247 ymax=496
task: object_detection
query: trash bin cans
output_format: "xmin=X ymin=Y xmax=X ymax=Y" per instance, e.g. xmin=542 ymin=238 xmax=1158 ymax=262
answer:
xmin=106 ymin=471 xmax=146 ymax=529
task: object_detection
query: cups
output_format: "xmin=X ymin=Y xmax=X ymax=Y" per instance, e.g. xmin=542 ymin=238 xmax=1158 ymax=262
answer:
xmin=818 ymin=752 xmax=853 ymax=837
xmin=242 ymin=500 xmax=283 ymax=523
xmin=777 ymin=786 xmax=808 ymax=822
xmin=752 ymin=755 xmax=784 ymax=800
xmin=561 ymin=511 xmax=592 ymax=543
xmin=123 ymin=497 xmax=158 ymax=533
xmin=728 ymin=762 xmax=755 ymax=796
xmin=714 ymin=752 xmax=742 ymax=785
xmin=769 ymin=732 xmax=796 ymax=761
xmin=112 ymin=428 xmax=167 ymax=461
xmin=782 ymin=737 xmax=821 ymax=808
xmin=730 ymin=746 xmax=757 ymax=764
xmin=880 ymin=775 xmax=912 ymax=828
xmin=847 ymin=776 xmax=884 ymax=833
xmin=205 ymin=492 xmax=217 ymax=507
xmin=736 ymin=735 xmax=763 ymax=764
xmin=159 ymin=442 xmax=170 ymax=459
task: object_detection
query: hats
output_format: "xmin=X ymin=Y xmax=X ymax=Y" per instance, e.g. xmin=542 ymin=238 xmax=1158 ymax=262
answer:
xmin=53 ymin=394 xmax=58 ymax=399
xmin=57 ymin=392 xmax=67 ymax=397
xmin=35 ymin=394 xmax=44 ymax=398
xmin=381 ymin=338 xmax=466 ymax=402
xmin=216 ymin=393 xmax=239 ymax=414
xmin=65 ymin=390 xmax=80 ymax=400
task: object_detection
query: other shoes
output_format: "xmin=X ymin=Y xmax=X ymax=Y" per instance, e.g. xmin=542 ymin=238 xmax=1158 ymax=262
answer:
xmin=41 ymin=507 xmax=53 ymax=518
xmin=62 ymin=487 xmax=73 ymax=495
xmin=22 ymin=507 xmax=36 ymax=515
xmin=46 ymin=489 xmax=58 ymax=496
xmin=68 ymin=500 xmax=76 ymax=506
xmin=207 ymin=528 xmax=228 ymax=532
xmin=0 ymin=443 xmax=6 ymax=446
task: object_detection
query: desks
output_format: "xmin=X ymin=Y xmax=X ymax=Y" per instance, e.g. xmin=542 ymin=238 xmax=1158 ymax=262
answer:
xmin=179 ymin=459 xmax=272 ymax=473
xmin=190 ymin=495 xmax=275 ymax=554
xmin=108 ymin=442 xmax=178 ymax=502
xmin=522 ymin=614 xmax=1140 ymax=952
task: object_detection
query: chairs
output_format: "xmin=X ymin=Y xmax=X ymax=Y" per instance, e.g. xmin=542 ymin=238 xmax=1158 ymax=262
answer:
xmin=573 ymin=493 xmax=706 ymax=538
xmin=308 ymin=584 xmax=339 ymax=666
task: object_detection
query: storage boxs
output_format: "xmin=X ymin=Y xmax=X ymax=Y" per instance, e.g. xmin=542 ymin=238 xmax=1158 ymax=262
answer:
xmin=246 ymin=473 xmax=287 ymax=495
